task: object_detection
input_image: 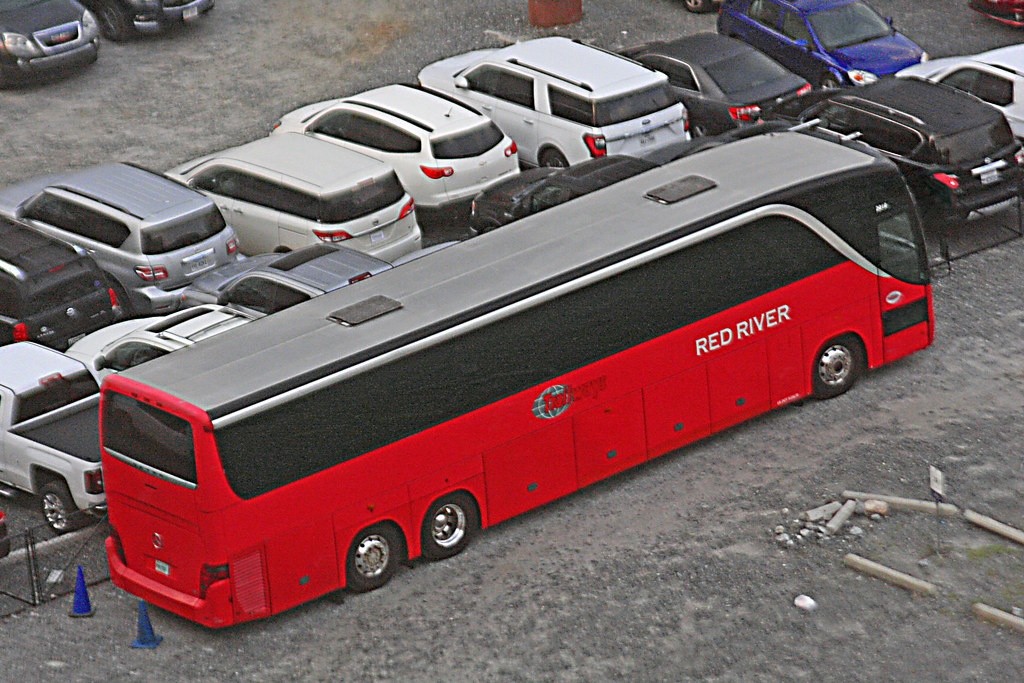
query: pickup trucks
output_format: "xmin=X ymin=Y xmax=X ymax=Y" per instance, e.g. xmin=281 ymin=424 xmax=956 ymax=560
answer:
xmin=1 ymin=340 xmax=110 ymax=532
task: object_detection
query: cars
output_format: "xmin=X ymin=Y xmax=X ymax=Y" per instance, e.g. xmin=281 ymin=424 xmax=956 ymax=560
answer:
xmin=895 ymin=41 xmax=1024 ymax=139
xmin=969 ymin=0 xmax=1024 ymax=29
xmin=82 ymin=0 xmax=216 ymax=41
xmin=162 ymin=130 xmax=423 ymax=263
xmin=0 ymin=508 xmax=12 ymax=559
xmin=716 ymin=0 xmax=929 ymax=90
xmin=673 ymin=0 xmax=720 ymax=13
xmin=610 ymin=31 xmax=817 ymax=137
xmin=0 ymin=0 xmax=100 ymax=88
xmin=470 ymin=118 xmax=791 ymax=235
xmin=773 ymin=74 xmax=1024 ymax=223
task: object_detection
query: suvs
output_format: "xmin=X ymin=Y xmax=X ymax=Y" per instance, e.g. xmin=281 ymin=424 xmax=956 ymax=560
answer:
xmin=267 ymin=82 xmax=521 ymax=222
xmin=0 ymin=212 xmax=129 ymax=350
xmin=178 ymin=241 xmax=390 ymax=320
xmin=63 ymin=304 xmax=256 ymax=392
xmin=414 ymin=33 xmax=692 ymax=169
xmin=0 ymin=160 xmax=247 ymax=314
xmin=94 ymin=111 xmax=938 ymax=633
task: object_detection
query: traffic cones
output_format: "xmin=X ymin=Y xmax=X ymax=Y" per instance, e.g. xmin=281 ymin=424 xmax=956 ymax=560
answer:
xmin=128 ymin=598 xmax=163 ymax=649
xmin=66 ymin=560 xmax=97 ymax=617
